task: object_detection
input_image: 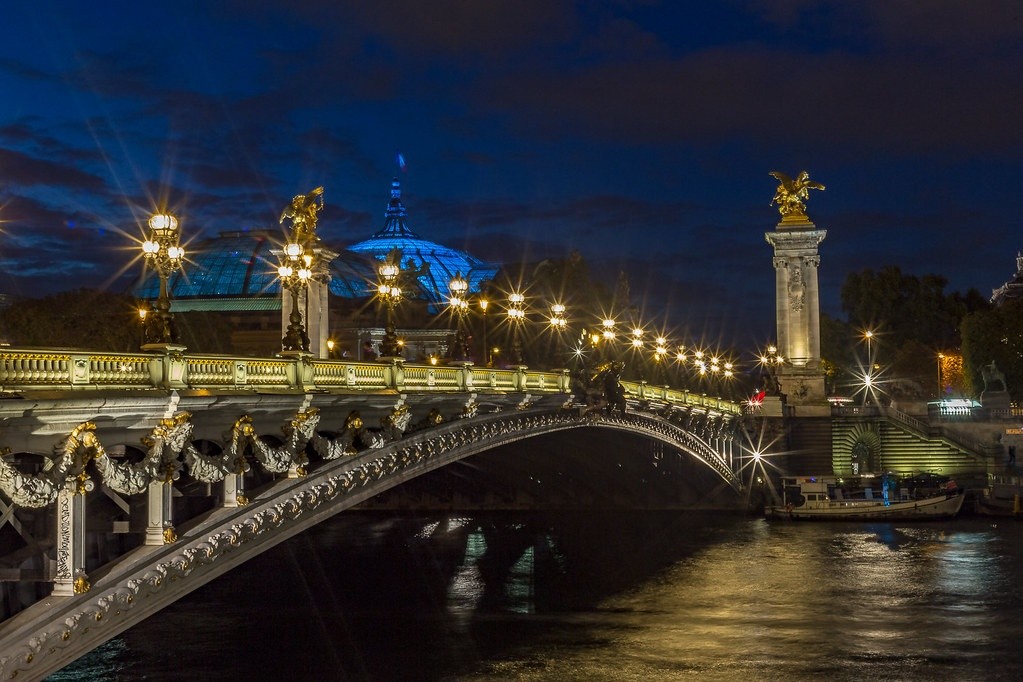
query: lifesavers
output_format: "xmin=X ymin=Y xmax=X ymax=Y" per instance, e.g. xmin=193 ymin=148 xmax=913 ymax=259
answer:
xmin=784 ymin=505 xmax=792 ymax=513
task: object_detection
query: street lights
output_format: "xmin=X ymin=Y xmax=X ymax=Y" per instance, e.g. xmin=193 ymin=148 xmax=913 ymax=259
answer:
xmin=506 ymin=291 xmax=527 ymax=366
xmin=141 ymin=207 xmax=180 ymax=345
xmin=548 ymin=304 xmax=568 ymax=370
xmin=278 ymin=241 xmax=313 ymax=352
xmin=938 ymin=353 xmax=944 ymax=399
xmin=477 ymin=295 xmax=491 ymax=366
xmin=447 ymin=272 xmax=472 ymax=362
xmin=866 ymin=331 xmax=873 ymax=373
xmin=373 ymin=248 xmax=404 ymax=358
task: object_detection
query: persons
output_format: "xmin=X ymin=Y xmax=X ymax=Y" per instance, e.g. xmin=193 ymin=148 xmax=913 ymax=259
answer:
xmin=362 ymin=341 xmax=378 ymax=363
xmin=1008 ymin=446 xmax=1017 ymax=465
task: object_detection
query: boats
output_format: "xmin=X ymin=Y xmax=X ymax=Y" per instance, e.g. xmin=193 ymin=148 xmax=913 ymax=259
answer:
xmin=765 ymin=470 xmax=968 ymax=523
xmin=982 ymin=479 xmax=1023 ymax=520
